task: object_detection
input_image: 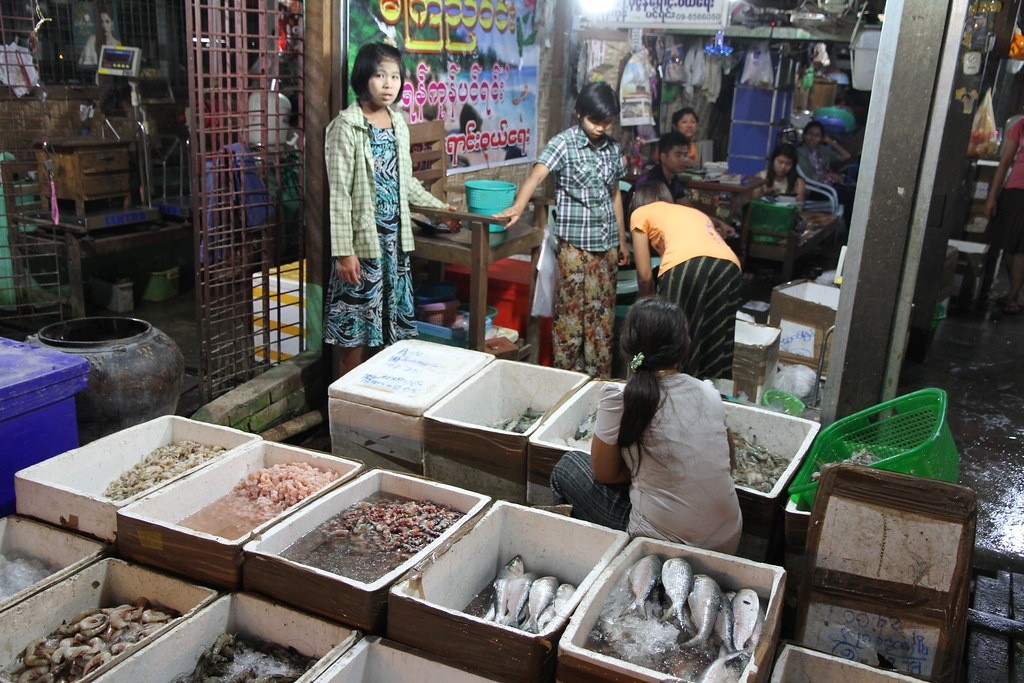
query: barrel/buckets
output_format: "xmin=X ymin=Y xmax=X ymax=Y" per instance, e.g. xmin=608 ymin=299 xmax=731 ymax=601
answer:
xmin=463 ymin=180 xmax=517 ymax=233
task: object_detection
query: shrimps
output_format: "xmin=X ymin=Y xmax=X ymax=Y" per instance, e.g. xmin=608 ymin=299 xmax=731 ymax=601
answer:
xmin=236 ymin=461 xmax=340 ymax=525
xmin=0 ymin=596 xmax=183 ymax=683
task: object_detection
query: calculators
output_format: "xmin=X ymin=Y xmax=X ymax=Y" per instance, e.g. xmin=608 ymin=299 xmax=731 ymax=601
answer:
xmin=410 ymin=217 xmax=451 ymax=234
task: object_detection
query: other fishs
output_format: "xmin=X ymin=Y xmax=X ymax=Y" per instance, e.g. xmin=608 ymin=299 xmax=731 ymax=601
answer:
xmin=102 ymin=404 xmax=790 ymax=683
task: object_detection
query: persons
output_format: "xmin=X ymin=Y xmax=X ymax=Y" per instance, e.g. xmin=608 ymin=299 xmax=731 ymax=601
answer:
xmin=550 ymin=297 xmax=742 ymax=556
xmin=653 ymin=108 xmax=699 ymax=167
xmin=324 ymin=41 xmax=462 ymax=378
xmin=77 ymin=2 xmax=122 ymax=64
xmin=493 ymin=82 xmax=631 ymax=380
xmin=627 ymin=178 xmax=741 ymax=381
xmin=753 ymin=143 xmax=805 ymax=210
xmin=984 ymin=117 xmax=1024 ymax=314
xmin=622 ymin=131 xmax=735 ymax=239
xmin=796 ymin=121 xmax=851 ymax=211
xmin=961 ymin=94 xmax=971 ymax=114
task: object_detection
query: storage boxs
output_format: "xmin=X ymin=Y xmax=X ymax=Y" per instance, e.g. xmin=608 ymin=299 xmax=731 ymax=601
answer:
xmin=0 ymin=160 xmax=1001 ymax=683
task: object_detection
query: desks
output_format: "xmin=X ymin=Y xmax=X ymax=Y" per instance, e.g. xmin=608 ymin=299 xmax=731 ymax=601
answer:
xmin=747 ymin=203 xmax=847 ymax=284
xmin=618 ymin=162 xmax=768 ymax=272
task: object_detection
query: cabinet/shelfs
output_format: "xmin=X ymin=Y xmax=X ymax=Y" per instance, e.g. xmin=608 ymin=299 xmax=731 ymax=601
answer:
xmin=404 ymin=196 xmax=550 ymax=363
xmin=30 ymin=137 xmax=136 ymax=219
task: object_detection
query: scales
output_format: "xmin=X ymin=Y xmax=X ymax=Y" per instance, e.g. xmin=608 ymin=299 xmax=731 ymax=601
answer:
xmin=123 ymin=75 xmax=193 ymax=221
xmin=47 ymin=44 xmax=161 ymax=230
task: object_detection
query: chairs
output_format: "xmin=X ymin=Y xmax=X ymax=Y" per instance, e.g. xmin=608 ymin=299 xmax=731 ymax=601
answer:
xmin=782 ymin=126 xmax=840 ymax=215
